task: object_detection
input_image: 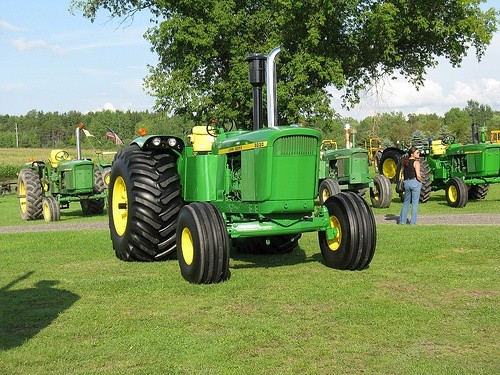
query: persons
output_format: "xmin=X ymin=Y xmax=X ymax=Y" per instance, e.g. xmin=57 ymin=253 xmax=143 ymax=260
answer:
xmin=398 ymin=147 xmax=422 ymax=224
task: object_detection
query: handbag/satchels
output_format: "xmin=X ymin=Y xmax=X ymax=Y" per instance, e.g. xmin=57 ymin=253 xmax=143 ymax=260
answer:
xmin=396 ymin=179 xmax=405 ymax=193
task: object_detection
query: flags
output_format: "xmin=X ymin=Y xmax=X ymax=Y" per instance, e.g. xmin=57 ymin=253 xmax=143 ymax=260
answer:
xmin=83 ymin=129 xmax=102 ymax=144
xmin=106 ymin=127 xmax=123 ymax=145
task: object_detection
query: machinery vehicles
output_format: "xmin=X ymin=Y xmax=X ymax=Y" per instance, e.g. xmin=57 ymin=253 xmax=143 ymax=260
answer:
xmin=108 ymin=49 xmax=500 ymax=285
xmin=16 ymin=122 xmax=108 ymax=221
